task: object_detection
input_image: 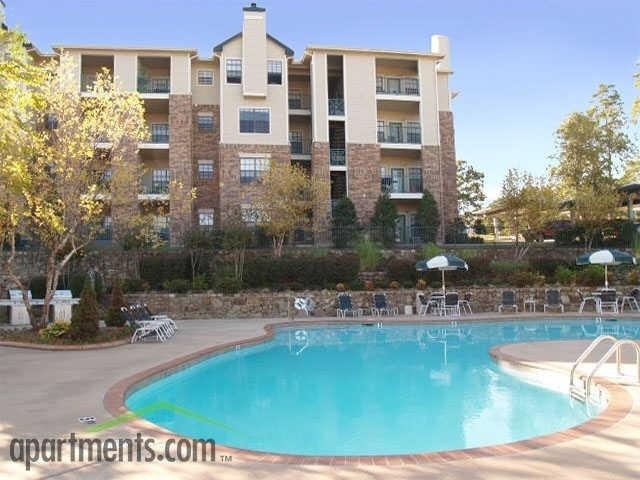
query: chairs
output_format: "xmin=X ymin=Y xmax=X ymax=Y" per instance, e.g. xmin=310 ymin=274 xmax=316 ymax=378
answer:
xmin=332 ymin=287 xmax=640 ymax=321
xmin=339 ymin=321 xmax=640 ymax=350
xmin=120 ymin=303 xmax=178 ymax=345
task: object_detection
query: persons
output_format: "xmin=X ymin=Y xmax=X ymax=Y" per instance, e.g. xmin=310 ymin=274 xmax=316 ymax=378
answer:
xmin=335 ymin=283 xmax=353 ymax=316
xmin=412 ymin=278 xmax=432 ymax=314
xmin=359 ymin=279 xmax=403 ymax=316
xmin=463 ymin=288 xmax=472 ymax=312
xmin=567 ymin=268 xmax=640 ymax=313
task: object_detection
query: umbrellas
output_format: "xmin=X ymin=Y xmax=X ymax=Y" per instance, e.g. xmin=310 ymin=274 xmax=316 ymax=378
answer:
xmin=415 ymin=252 xmax=469 ymax=294
xmin=575 ymin=248 xmax=637 ymax=289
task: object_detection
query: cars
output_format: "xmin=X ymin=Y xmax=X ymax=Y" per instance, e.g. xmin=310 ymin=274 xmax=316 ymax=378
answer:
xmin=523 ymin=219 xmax=640 ymax=248
